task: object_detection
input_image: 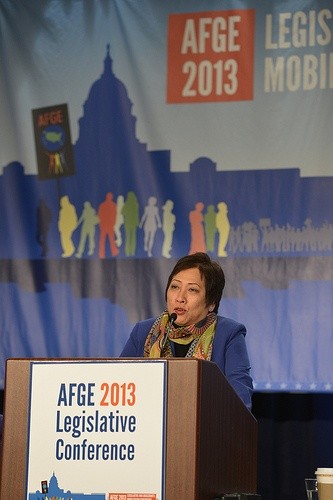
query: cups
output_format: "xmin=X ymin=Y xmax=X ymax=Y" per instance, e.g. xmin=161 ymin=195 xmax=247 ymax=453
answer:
xmin=315 ymin=468 xmax=333 ymax=500
xmin=305 ymin=479 xmax=318 ymax=500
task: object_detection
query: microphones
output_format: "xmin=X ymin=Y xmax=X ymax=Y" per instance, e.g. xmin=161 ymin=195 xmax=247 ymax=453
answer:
xmin=160 ymin=313 xmax=177 ymax=357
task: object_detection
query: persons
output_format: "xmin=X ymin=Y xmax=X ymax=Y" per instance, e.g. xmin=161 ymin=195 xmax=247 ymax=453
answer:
xmin=118 ymin=252 xmax=255 ymax=412
